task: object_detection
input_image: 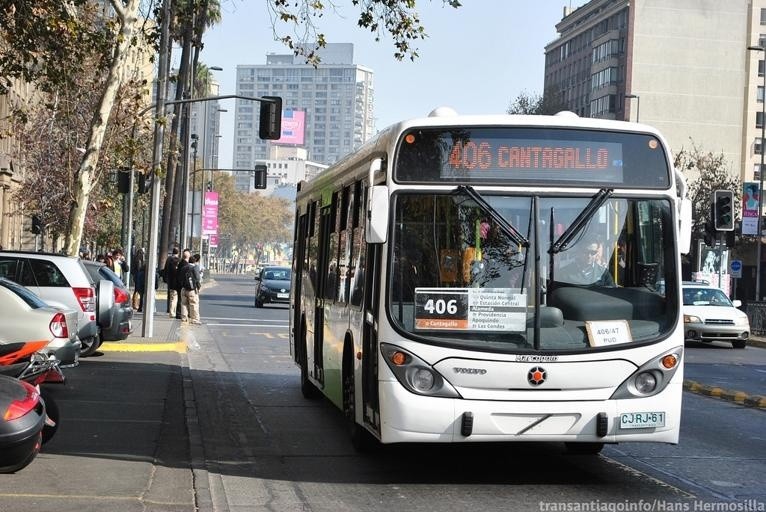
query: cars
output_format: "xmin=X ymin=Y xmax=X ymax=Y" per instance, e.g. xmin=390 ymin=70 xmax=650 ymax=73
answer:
xmin=0 ymin=276 xmax=82 ymax=370
xmin=657 ymin=279 xmax=751 ymax=349
xmin=254 ymin=266 xmax=293 ymax=309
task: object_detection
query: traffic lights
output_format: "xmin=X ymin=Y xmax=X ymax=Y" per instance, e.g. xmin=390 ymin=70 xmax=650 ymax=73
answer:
xmin=714 ymin=189 xmax=735 ymax=233
xmin=138 ymin=171 xmax=152 ymax=195
xmin=703 ymin=219 xmax=717 ymax=249
xmin=725 ymin=222 xmax=741 ymax=248
xmin=31 ymin=215 xmax=43 ymax=234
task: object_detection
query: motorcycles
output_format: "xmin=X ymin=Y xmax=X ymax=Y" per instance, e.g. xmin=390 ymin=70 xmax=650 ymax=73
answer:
xmin=0 ymin=339 xmax=66 ymax=474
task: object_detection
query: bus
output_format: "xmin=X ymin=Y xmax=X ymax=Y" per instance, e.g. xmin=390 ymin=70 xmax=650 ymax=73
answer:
xmin=287 ymin=105 xmax=686 ymax=465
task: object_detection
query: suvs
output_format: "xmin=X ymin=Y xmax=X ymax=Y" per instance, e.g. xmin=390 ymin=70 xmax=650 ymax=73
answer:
xmin=0 ymin=249 xmax=134 ymax=358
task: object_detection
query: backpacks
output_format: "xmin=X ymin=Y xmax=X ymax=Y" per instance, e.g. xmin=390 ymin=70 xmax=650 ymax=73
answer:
xmin=183 ymin=267 xmax=198 ymax=292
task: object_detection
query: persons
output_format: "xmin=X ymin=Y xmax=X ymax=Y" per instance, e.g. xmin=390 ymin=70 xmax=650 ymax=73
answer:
xmin=552 ymin=235 xmax=616 ymax=289
xmin=82 ymin=248 xmax=203 ymax=326
xmin=746 ymin=186 xmax=758 ymax=211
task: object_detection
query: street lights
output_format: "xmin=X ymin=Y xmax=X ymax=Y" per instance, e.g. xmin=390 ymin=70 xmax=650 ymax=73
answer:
xmin=624 ymin=93 xmax=640 ymax=123
xmin=183 ymin=65 xmax=227 ymax=276
xmin=747 ymin=45 xmax=766 ymax=304
